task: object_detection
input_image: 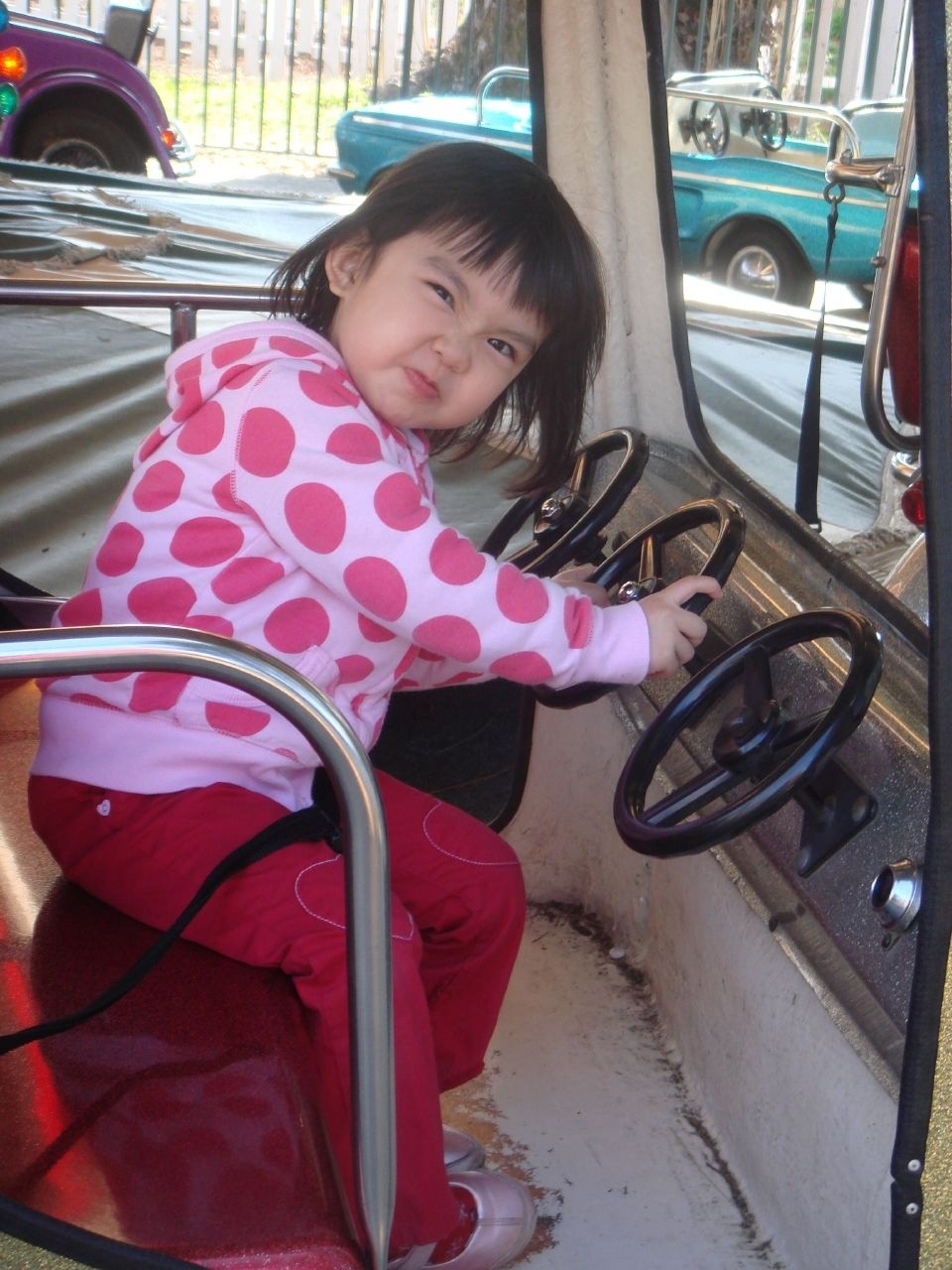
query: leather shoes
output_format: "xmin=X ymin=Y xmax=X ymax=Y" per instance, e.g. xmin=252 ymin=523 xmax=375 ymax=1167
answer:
xmin=442 ymin=1127 xmax=488 ymax=1173
xmin=387 ymin=1170 xmax=537 ymax=1270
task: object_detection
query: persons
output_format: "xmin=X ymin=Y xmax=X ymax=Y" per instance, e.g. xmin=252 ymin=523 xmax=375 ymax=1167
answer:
xmin=26 ymin=143 xmax=724 ymax=1270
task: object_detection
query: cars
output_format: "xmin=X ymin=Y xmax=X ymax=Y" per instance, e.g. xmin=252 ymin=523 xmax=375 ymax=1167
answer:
xmin=327 ymin=62 xmax=922 ymax=312
xmin=0 ymin=0 xmax=196 ymax=184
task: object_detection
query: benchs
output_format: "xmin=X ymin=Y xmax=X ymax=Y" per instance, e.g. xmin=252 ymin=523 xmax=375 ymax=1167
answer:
xmin=0 ymin=586 xmax=400 ymax=1270
